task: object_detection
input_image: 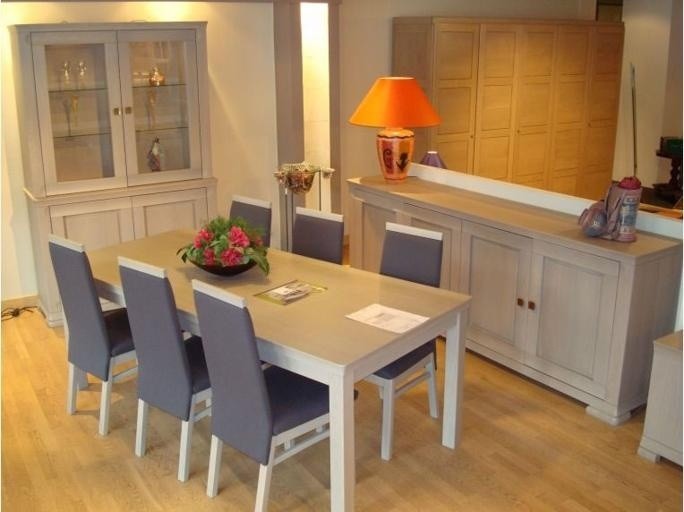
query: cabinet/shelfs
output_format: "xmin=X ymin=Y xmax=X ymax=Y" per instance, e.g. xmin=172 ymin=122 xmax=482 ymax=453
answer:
xmin=8 ymin=22 xmax=212 ymax=198
xmin=392 ymin=17 xmax=625 ymax=201
xmin=637 ymin=330 xmax=683 ymax=467
xmin=23 ymin=178 xmax=218 ymax=328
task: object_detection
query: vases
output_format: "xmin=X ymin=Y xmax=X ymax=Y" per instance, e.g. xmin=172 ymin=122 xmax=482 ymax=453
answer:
xmin=189 ymin=259 xmax=257 ymax=276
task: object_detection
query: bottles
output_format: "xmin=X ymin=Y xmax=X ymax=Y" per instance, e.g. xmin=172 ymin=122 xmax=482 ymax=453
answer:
xmin=148 ymin=139 xmax=167 ymax=171
xmin=76 ymin=60 xmax=86 ymax=89
xmin=58 ymin=61 xmax=75 ymax=89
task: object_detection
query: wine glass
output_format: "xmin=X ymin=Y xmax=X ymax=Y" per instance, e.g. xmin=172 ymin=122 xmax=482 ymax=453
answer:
xmin=70 ymin=98 xmax=80 ymax=136
xmin=147 ymin=90 xmax=161 ymax=130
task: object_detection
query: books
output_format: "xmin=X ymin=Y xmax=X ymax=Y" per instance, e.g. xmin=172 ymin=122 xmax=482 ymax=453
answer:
xmin=253 ymin=280 xmax=327 ymax=308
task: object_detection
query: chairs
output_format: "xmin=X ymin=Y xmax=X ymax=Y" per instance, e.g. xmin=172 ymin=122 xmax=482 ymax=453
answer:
xmin=316 ymin=222 xmax=443 ymax=461
xmin=230 ymin=197 xmax=271 ymax=247
xmin=119 ymin=257 xmax=266 ymax=482
xmin=292 ymin=207 xmax=344 ymax=265
xmin=48 ymin=236 xmax=138 ymax=436
xmin=190 ymin=279 xmax=358 ymax=512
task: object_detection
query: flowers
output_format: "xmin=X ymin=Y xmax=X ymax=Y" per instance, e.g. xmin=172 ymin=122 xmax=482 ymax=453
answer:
xmin=177 ymin=217 xmax=269 ymax=276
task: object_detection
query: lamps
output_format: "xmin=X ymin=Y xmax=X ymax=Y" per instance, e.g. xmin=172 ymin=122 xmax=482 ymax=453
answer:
xmin=419 ymin=151 xmax=447 ymax=169
xmin=349 ymin=77 xmax=442 ymax=180
